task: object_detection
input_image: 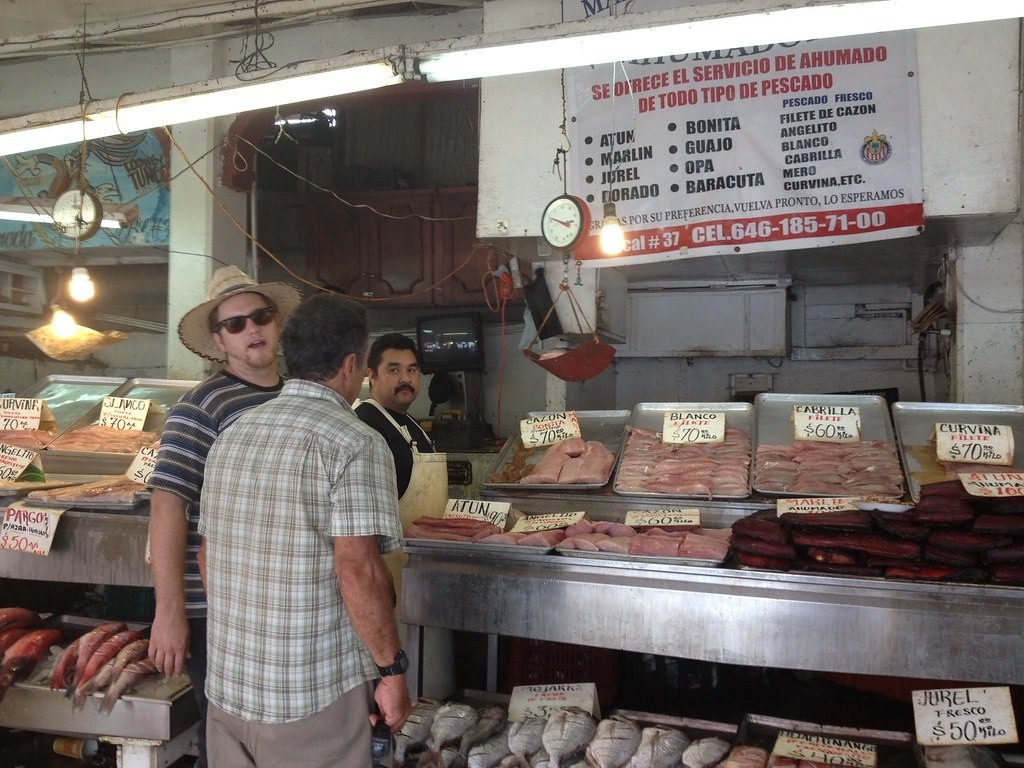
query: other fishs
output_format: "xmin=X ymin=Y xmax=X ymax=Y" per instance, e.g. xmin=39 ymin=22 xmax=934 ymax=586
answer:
xmin=1 ymin=608 xmax=164 ymax=713
xmin=392 ymin=697 xmax=730 ymax=767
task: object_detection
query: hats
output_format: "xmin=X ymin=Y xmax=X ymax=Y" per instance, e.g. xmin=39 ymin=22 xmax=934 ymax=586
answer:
xmin=177 ymin=265 xmax=304 ymax=363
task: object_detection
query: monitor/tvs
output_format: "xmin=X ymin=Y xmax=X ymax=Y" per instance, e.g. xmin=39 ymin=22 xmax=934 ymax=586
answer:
xmin=416 ymin=312 xmax=485 ymax=375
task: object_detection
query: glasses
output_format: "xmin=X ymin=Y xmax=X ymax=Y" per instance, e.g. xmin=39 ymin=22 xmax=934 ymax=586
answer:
xmin=210 ymin=306 xmax=276 ymax=334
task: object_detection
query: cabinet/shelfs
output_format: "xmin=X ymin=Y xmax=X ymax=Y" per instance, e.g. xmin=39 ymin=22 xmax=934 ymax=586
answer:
xmin=617 ymin=288 xmax=790 ymax=358
xmin=305 ymin=185 xmax=545 ymax=309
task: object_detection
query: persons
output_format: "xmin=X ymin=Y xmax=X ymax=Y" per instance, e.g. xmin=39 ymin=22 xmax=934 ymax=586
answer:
xmin=146 ymin=266 xmax=300 ymax=681
xmin=196 ymin=293 xmax=412 ymax=768
xmin=353 ymin=334 xmax=451 ymax=700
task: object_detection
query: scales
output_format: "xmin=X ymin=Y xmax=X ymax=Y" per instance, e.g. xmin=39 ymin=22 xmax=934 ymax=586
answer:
xmin=24 ymin=186 xmax=127 ymax=361
xmin=522 ymin=195 xmax=616 ymax=381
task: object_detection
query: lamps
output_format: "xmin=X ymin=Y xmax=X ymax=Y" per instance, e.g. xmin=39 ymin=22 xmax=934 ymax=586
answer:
xmin=1 ymin=194 xmax=140 ymax=232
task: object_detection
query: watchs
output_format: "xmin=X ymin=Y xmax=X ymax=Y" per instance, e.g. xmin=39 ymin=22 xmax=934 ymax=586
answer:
xmin=376 ymin=650 xmax=409 ymax=676
